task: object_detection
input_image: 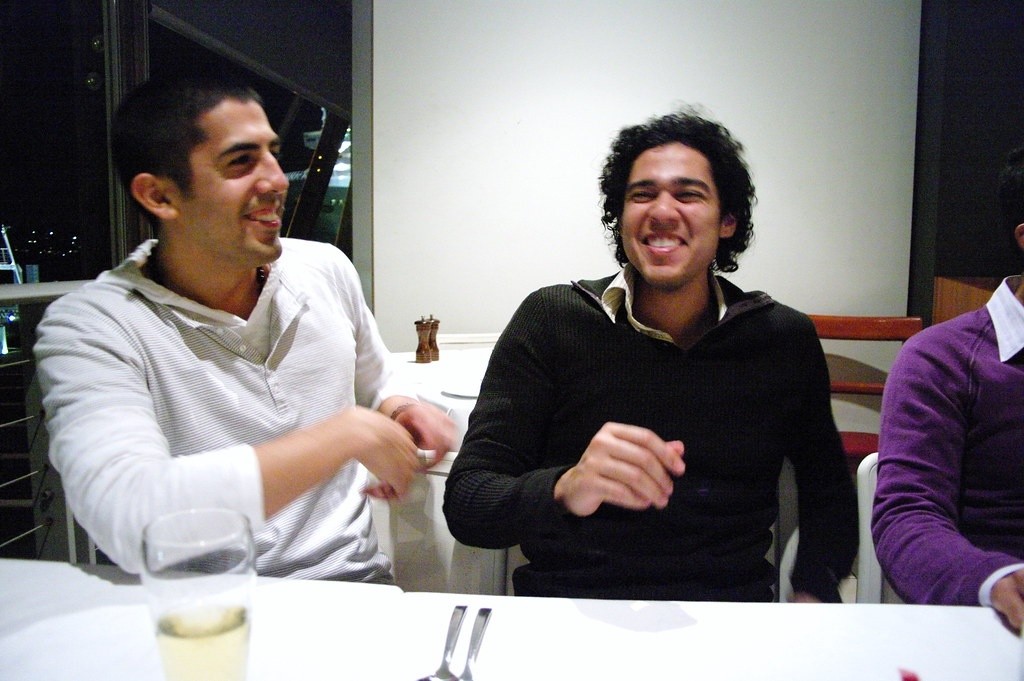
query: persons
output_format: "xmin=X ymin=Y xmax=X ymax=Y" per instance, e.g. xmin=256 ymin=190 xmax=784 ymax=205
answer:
xmin=441 ymin=113 xmax=860 ymax=603
xmin=32 ymin=59 xmax=459 ymax=588
xmin=870 ymin=147 xmax=1024 ymax=632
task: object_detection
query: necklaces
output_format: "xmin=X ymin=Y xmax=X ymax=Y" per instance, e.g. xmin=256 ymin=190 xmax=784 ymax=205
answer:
xmin=257 ymin=267 xmax=266 ymax=293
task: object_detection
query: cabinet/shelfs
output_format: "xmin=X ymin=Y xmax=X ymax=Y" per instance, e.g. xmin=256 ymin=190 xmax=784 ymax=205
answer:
xmin=932 ymin=276 xmax=1000 ymax=327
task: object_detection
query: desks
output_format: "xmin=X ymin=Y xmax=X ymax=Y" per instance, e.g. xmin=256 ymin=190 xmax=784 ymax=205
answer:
xmin=363 ymin=350 xmax=528 ymax=595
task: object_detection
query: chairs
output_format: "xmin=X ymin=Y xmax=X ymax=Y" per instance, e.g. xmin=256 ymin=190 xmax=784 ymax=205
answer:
xmin=857 ymin=452 xmax=907 ymax=604
xmin=811 ymin=316 xmax=923 ymax=457
xmin=479 ymin=455 xmax=798 ymax=602
xmin=371 ymin=455 xmax=493 ymax=595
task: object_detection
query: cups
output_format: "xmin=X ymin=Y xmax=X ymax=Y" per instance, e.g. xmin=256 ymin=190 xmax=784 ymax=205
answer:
xmin=140 ymin=507 xmax=258 ymax=681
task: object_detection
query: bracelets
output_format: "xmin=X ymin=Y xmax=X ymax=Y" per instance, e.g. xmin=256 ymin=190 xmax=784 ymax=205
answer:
xmin=392 ymin=403 xmax=416 ymax=420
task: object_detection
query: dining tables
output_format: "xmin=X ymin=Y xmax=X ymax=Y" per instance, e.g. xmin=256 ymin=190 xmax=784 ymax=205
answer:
xmin=0 ymin=559 xmax=1024 ymax=681
